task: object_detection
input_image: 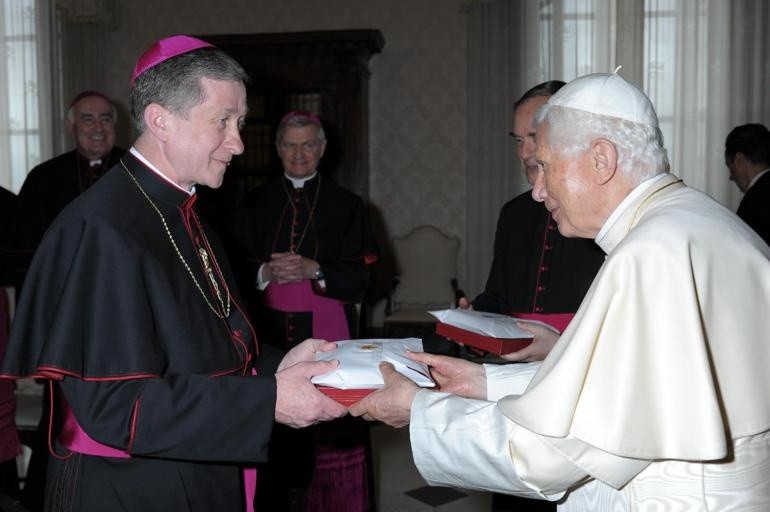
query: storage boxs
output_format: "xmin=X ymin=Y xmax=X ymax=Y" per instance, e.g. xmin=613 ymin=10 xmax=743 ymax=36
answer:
xmin=315 ymin=384 xmax=441 ymax=414
xmin=434 ymin=318 xmax=534 ymax=357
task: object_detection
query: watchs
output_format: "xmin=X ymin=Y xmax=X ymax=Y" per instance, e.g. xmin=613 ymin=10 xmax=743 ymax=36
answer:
xmin=311 ymin=261 xmax=324 ymax=278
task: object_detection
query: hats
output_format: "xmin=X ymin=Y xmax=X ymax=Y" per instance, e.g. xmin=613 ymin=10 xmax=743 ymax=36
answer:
xmin=546 ymin=64 xmax=660 ymax=129
xmin=68 ymin=90 xmax=112 ymax=110
xmin=280 ymin=109 xmax=322 ymax=127
xmin=126 ymin=32 xmax=216 ymax=96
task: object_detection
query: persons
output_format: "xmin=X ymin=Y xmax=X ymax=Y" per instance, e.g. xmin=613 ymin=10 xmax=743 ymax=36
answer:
xmin=0 ymin=35 xmax=349 ymax=511
xmin=19 ymin=89 xmax=126 ymax=512
xmin=445 ymin=79 xmax=606 ymax=364
xmin=348 ymin=65 xmax=770 ymax=511
xmin=222 ymin=106 xmax=399 ymax=511
xmin=724 ymin=123 xmax=769 ymax=246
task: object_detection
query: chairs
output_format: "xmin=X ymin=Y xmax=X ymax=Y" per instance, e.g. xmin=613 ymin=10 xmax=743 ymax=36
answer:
xmin=381 ymin=224 xmax=465 ymax=338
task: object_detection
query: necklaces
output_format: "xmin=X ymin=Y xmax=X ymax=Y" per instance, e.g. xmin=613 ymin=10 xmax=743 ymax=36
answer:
xmin=278 ymin=172 xmax=322 ymax=255
xmin=118 ymin=159 xmax=231 ymax=318
